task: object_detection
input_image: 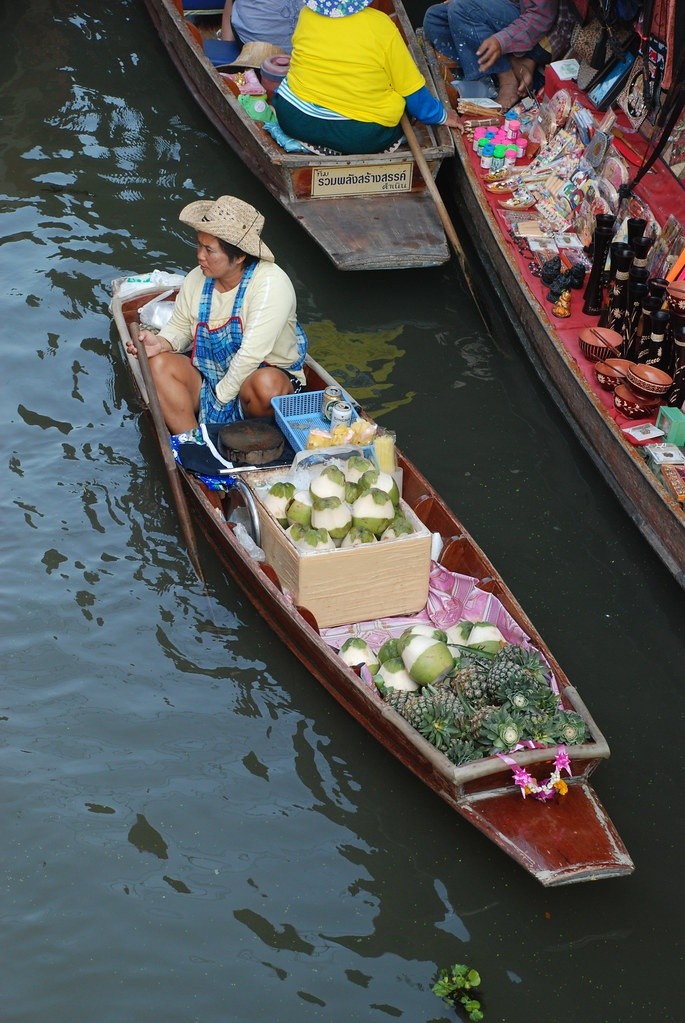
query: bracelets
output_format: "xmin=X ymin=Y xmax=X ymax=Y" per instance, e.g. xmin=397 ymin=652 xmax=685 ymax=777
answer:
xmin=509 ymin=231 xmax=541 ymax=277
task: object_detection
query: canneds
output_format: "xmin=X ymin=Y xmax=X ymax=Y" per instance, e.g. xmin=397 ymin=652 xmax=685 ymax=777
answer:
xmin=321 ymin=386 xmax=342 ymax=423
xmin=331 ymin=401 xmax=352 ymax=431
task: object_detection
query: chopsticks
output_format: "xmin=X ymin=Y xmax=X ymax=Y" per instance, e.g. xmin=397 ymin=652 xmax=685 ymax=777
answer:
xmin=590 ymin=353 xmax=627 ymax=378
xmin=590 ymin=329 xmax=621 ymax=357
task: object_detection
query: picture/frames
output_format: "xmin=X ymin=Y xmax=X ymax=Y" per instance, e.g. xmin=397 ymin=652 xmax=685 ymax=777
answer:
xmin=586 ymin=51 xmax=637 ymax=112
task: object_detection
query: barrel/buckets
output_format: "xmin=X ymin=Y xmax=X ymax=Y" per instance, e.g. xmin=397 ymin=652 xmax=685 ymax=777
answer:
xmin=260 ymin=55 xmax=291 ymax=105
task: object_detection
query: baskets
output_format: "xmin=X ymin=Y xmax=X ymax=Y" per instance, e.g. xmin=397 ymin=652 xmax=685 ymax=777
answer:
xmin=271 ymin=389 xmax=375 ymax=466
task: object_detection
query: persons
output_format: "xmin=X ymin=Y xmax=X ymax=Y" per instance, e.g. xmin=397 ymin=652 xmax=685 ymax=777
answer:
xmin=273 ymin=0 xmax=465 ymax=155
xmin=215 ymin=0 xmax=306 ymax=56
xmin=125 ymin=195 xmax=311 ymax=436
xmin=423 ymin=0 xmax=559 ymax=114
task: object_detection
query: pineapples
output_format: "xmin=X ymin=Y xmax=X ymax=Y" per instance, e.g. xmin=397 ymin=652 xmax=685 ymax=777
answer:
xmin=383 ymin=643 xmax=589 ymax=765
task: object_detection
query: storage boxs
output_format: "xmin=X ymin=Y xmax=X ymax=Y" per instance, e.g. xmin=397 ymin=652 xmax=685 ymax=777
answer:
xmin=240 ymin=471 xmax=433 ymax=628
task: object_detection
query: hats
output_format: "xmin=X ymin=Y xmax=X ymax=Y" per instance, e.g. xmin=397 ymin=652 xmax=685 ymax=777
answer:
xmin=303 ymin=0 xmax=373 ymax=18
xmin=215 ymin=41 xmax=287 ymax=70
xmin=571 ymin=19 xmax=637 ymax=92
xmin=178 ymin=194 xmax=276 ymax=263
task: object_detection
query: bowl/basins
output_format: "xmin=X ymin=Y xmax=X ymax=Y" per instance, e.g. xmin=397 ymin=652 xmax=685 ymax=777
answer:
xmin=613 ymin=384 xmax=660 ymax=419
xmin=626 ymin=363 xmax=673 ymax=402
xmin=594 ymin=359 xmax=637 ymax=392
xmin=578 ymin=327 xmax=624 ymax=361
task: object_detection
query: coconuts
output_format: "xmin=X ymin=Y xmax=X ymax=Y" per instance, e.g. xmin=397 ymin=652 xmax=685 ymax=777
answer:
xmin=264 ymin=455 xmax=414 ymax=552
xmin=338 ymin=620 xmax=510 ymax=695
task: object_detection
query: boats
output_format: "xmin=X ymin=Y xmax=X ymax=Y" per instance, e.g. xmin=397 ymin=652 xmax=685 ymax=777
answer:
xmin=146 ymin=0 xmax=453 ymax=270
xmin=107 ymin=267 xmax=636 ymax=887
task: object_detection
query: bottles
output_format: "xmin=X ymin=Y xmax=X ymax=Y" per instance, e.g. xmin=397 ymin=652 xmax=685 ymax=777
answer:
xmin=581 ymin=214 xmax=685 ymax=408
